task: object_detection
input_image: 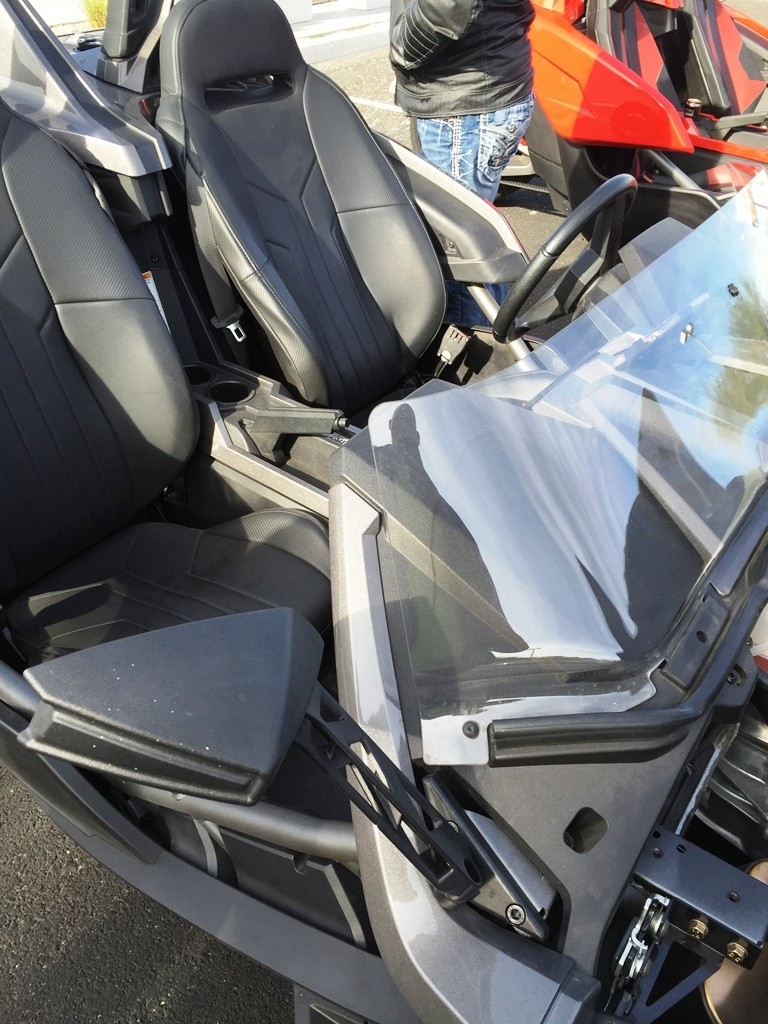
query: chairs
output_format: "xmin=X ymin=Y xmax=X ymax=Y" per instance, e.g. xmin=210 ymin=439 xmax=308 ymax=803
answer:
xmin=0 ymin=96 xmax=332 ymax=671
xmin=153 ymin=0 xmax=568 ymax=430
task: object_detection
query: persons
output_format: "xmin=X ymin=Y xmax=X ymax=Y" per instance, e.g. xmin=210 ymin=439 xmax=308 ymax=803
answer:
xmin=389 ymin=0 xmax=537 ymax=328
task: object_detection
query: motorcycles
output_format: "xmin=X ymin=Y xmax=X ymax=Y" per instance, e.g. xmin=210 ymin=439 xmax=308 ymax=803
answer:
xmin=0 ymin=0 xmax=767 ymax=1024
xmin=525 ymin=2 xmax=764 ymax=271
